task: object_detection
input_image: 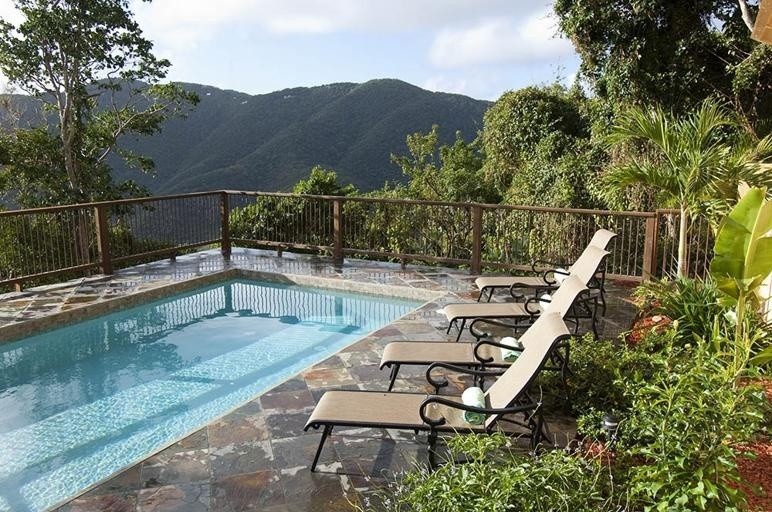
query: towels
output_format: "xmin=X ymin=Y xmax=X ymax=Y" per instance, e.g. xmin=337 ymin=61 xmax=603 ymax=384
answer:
xmin=461 ymin=387 xmax=486 ymax=425
xmin=500 ymin=337 xmax=521 ymax=362
xmin=539 ymin=294 xmax=553 ymax=312
xmin=554 ymin=268 xmax=570 ymax=284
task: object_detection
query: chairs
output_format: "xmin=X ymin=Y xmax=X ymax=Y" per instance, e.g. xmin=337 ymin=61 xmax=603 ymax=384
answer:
xmin=475 ymin=229 xmax=618 ymax=316
xmin=379 ymin=275 xmax=589 ymax=414
xmin=442 ymin=245 xmax=611 ymax=342
xmin=303 ymin=311 xmax=571 ymax=476
